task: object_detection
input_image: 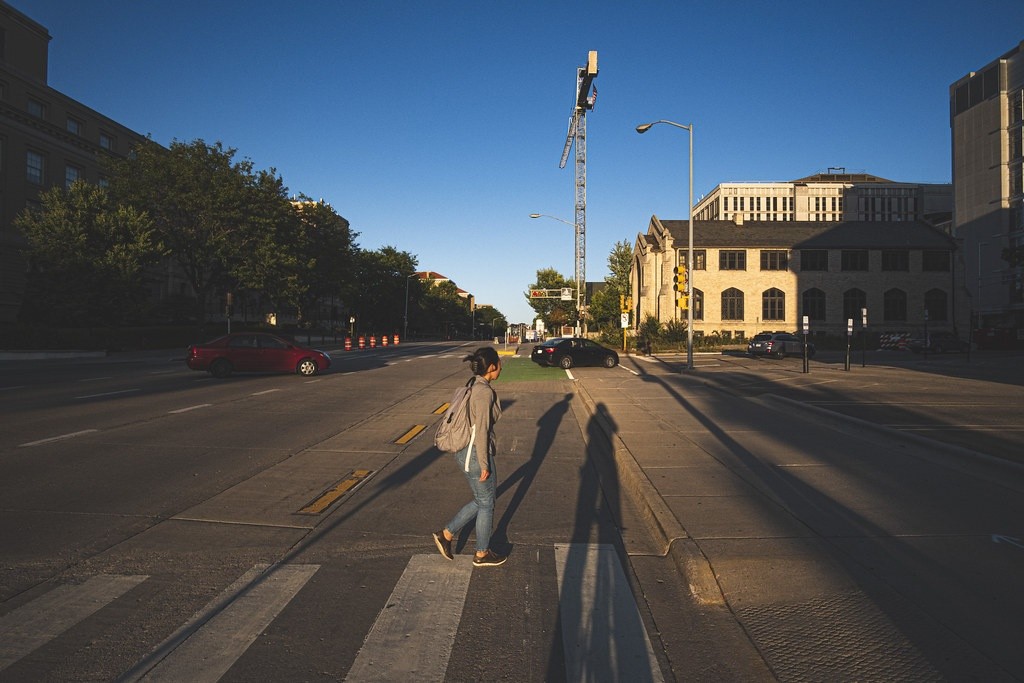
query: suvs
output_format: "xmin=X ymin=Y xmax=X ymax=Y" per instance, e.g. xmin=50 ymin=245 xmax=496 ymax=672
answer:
xmin=747 ymin=332 xmax=816 ymax=360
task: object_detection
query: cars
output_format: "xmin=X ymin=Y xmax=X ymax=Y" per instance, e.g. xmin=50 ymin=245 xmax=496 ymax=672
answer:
xmin=532 ymin=338 xmax=619 ymax=368
xmin=186 ymin=332 xmax=331 ymax=379
xmin=912 ymin=328 xmax=969 ymax=354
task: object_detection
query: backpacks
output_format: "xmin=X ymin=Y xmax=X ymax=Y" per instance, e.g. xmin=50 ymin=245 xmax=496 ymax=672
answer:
xmin=433 ymin=377 xmax=497 ymax=453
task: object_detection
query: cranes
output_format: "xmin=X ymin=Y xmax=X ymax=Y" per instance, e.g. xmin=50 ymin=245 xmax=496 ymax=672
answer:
xmin=558 ymin=51 xmax=599 ymax=340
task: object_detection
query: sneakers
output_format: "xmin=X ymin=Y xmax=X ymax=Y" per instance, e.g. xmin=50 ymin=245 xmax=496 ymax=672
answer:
xmin=433 ymin=530 xmax=454 ymax=560
xmin=473 ymin=548 xmax=507 ymax=567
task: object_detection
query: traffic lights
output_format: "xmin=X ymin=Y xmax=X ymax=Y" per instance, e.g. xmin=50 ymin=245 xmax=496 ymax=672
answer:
xmin=675 ymin=298 xmax=687 ymax=307
xmin=673 ymin=266 xmax=685 ymax=293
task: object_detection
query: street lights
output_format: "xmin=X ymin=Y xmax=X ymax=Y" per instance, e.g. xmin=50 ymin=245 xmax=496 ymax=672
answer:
xmin=531 ymin=290 xmax=546 ymax=297
xmin=403 ymin=273 xmax=417 ymax=340
xmin=529 ymin=213 xmax=580 ymax=339
xmin=636 ymin=119 xmax=694 ymax=369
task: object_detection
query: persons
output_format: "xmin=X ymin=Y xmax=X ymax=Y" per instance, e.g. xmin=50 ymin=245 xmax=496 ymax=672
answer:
xmin=433 ymin=347 xmax=508 ymax=567
